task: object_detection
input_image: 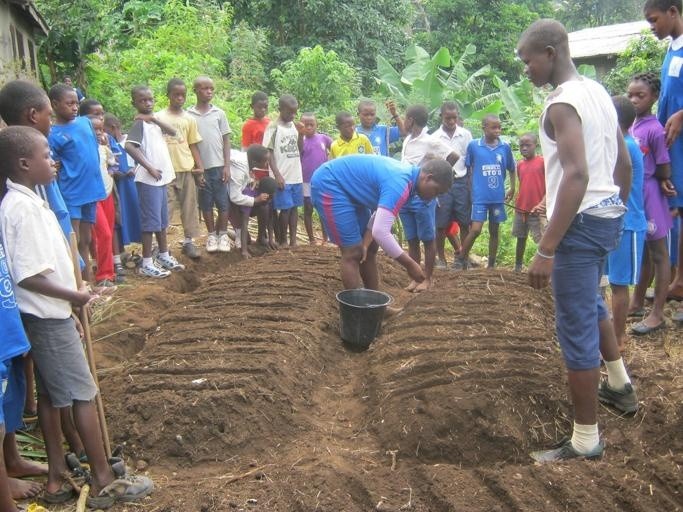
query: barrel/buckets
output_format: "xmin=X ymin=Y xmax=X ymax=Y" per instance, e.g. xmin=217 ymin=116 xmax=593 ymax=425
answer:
xmin=336 ymin=289 xmax=394 ymax=348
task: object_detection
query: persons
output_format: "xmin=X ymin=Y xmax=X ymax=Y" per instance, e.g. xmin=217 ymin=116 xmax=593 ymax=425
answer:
xmin=125 ymin=75 xmax=517 ymax=294
xmin=514 ymin=0 xmax=683 ymax=464
xmin=310 ymin=155 xmax=453 ymax=317
xmin=1 ymin=77 xmax=153 ymax=511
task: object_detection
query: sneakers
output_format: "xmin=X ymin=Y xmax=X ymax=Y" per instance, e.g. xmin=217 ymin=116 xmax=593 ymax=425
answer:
xmin=529 ymin=436 xmax=603 ymax=464
xmin=598 ymin=379 xmax=639 ymax=412
xmin=44 ymin=445 xmax=153 ymax=509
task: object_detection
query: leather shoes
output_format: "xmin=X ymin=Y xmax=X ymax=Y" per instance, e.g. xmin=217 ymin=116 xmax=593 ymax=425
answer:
xmin=625 ymin=288 xmax=683 ymax=333
